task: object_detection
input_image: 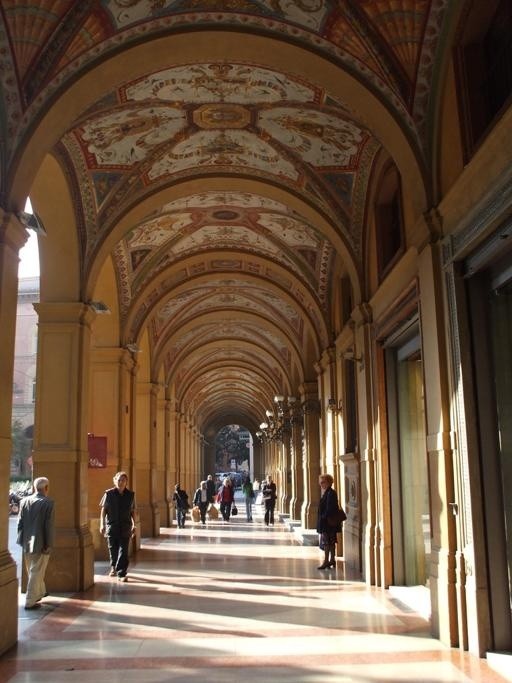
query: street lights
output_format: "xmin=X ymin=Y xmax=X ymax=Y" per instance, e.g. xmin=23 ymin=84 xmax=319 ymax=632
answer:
xmin=191 ymin=506 xmax=200 ymax=522
xmin=207 ymin=504 xmax=218 ymax=518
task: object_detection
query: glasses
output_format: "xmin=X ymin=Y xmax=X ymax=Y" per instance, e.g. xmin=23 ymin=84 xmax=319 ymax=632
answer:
xmin=16 ymin=210 xmax=48 ymax=237
xmin=125 ymin=343 xmax=143 ymax=353
xmin=256 ymin=395 xmax=321 ymax=448
xmin=88 ymin=300 xmax=112 ymax=314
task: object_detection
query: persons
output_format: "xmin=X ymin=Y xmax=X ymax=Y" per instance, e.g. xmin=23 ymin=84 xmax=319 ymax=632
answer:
xmin=16 ymin=477 xmax=57 ymax=610
xmin=316 ymin=473 xmax=347 ymax=570
xmin=172 ymin=474 xmax=277 ymax=529
xmin=99 ymin=472 xmax=137 ymax=582
